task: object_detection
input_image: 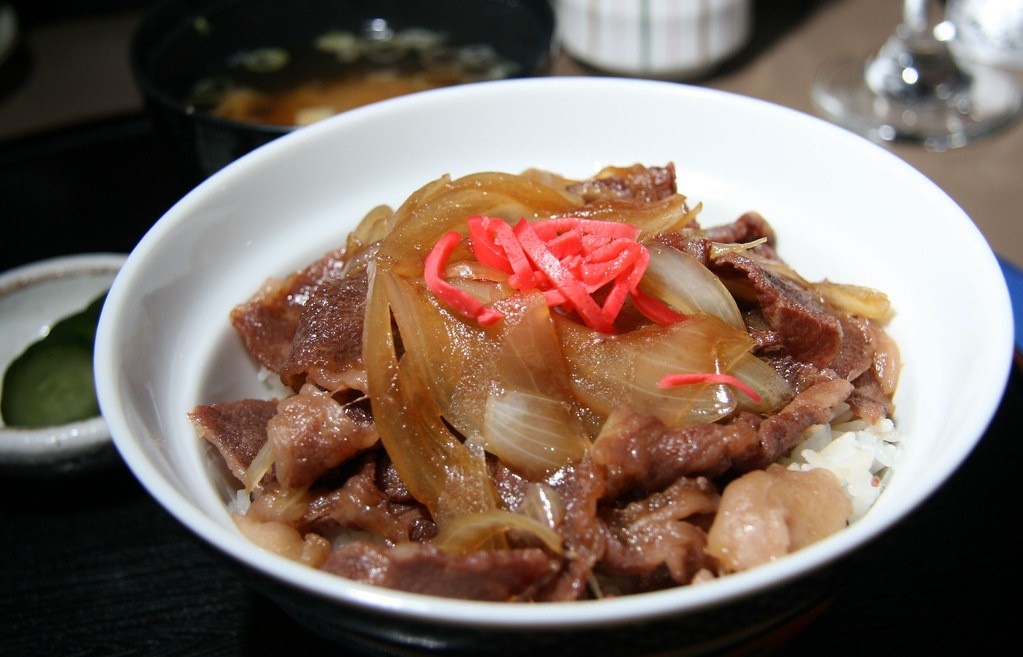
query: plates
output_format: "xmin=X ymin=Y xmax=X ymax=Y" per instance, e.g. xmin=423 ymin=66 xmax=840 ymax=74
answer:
xmin=94 ymin=79 xmax=1016 ymax=631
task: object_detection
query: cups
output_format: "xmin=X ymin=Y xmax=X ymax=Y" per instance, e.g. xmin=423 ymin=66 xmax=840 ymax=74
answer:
xmin=550 ymin=0 xmax=754 ymax=82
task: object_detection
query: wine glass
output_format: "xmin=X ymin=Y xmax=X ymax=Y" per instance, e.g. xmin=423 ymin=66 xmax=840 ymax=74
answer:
xmin=807 ymin=0 xmax=1021 ymax=142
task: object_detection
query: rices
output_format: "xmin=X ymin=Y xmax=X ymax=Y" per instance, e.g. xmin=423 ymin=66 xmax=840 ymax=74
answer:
xmin=234 ymin=359 xmax=900 ymax=526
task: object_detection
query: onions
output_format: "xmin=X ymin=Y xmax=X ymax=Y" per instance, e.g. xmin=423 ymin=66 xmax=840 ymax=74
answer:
xmin=341 ymin=166 xmax=900 ymax=549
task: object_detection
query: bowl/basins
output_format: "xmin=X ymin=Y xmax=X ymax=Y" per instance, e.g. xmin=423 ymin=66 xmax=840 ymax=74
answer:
xmin=0 ymin=253 xmax=129 ymax=464
xmin=124 ymin=1 xmax=555 ymax=190
